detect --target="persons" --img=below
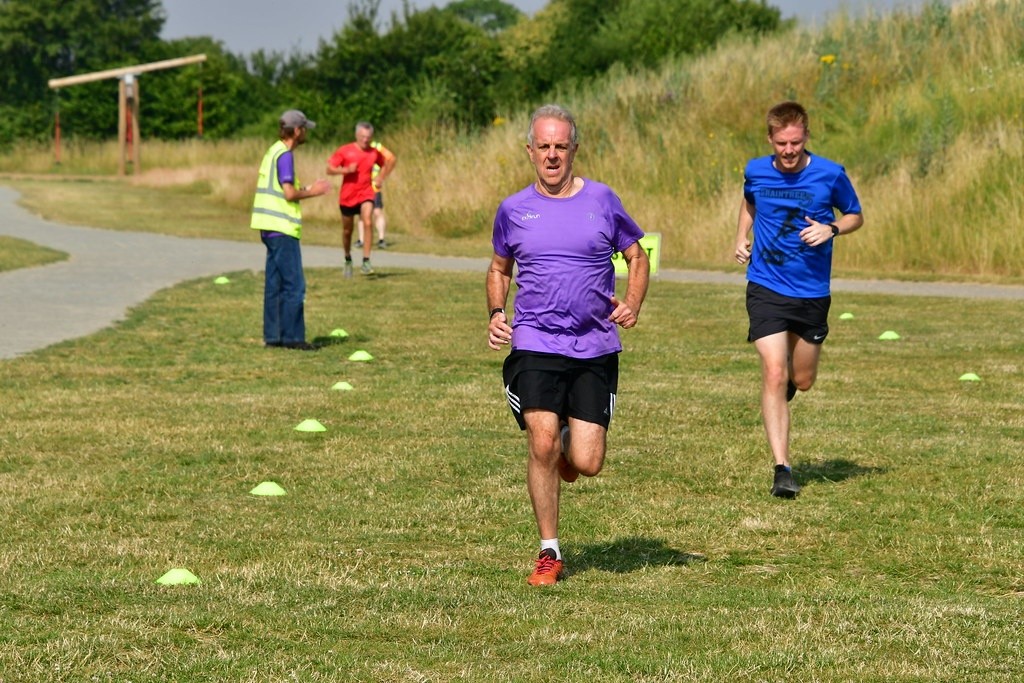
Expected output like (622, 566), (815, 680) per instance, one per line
(486, 104), (652, 587)
(734, 102), (865, 502)
(355, 141), (397, 248)
(326, 121), (386, 280)
(251, 110), (333, 352)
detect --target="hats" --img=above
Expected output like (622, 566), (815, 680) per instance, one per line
(280, 110), (315, 129)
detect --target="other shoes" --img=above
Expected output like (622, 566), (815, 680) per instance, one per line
(355, 240), (363, 247)
(360, 260), (374, 274)
(378, 239), (385, 249)
(282, 342), (321, 350)
(343, 261), (352, 279)
(266, 342), (282, 348)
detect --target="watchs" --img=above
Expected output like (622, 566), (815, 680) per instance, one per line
(827, 223), (839, 240)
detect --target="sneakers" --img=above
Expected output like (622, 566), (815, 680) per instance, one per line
(787, 378), (797, 401)
(528, 548), (563, 585)
(558, 419), (579, 482)
(770, 462), (799, 498)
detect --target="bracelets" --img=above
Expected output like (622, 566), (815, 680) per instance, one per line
(489, 307), (504, 320)
